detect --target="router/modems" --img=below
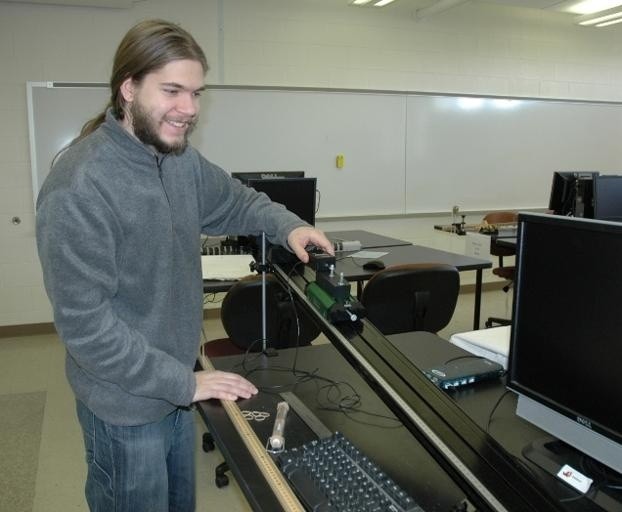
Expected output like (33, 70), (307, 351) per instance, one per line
(422, 358), (503, 391)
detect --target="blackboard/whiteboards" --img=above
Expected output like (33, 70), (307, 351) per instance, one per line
(24, 79), (622, 224)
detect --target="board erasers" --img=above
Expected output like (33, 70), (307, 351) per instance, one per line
(336, 153), (344, 170)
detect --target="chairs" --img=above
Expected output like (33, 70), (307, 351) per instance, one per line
(199, 275), (321, 361)
(362, 261), (460, 333)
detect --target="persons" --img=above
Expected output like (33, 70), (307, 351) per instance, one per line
(33, 17), (335, 511)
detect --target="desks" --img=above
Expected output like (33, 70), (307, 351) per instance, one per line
(307, 221), (514, 328)
(191, 328), (622, 512)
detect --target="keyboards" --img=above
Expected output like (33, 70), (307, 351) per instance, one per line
(273, 430), (425, 512)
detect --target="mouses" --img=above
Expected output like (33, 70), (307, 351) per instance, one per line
(363, 260), (386, 270)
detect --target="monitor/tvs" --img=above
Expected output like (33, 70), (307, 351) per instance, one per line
(504, 211), (622, 512)
(231, 172), (305, 189)
(592, 175), (622, 222)
(248, 176), (317, 229)
(548, 171), (600, 220)
